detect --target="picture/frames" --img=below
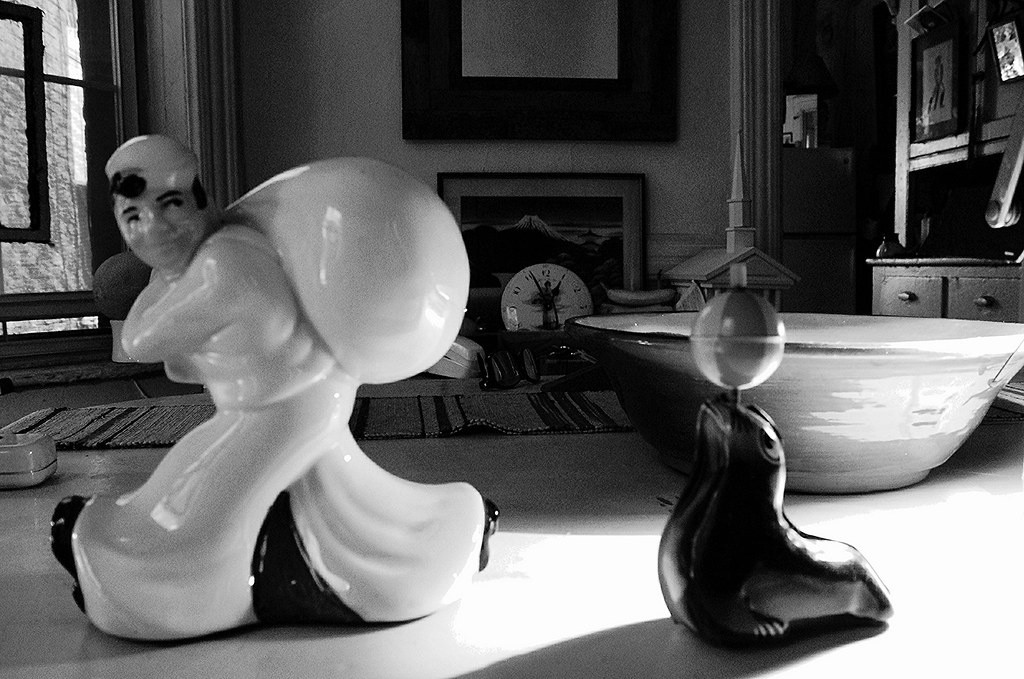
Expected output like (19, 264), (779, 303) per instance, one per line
(437, 170), (651, 338)
(908, 13), (973, 145)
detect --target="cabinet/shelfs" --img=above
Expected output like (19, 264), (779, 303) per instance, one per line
(866, 1), (1023, 384)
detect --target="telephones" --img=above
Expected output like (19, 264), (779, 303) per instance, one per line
(425, 335), (488, 379)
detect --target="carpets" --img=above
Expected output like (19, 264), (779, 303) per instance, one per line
(991, 380), (1023, 415)
(0, 390), (634, 453)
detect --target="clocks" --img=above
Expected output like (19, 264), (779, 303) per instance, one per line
(499, 262), (593, 331)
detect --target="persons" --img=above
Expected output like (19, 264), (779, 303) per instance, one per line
(48, 132), (503, 643)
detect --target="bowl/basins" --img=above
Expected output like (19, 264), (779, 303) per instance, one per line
(560, 309), (1024, 496)
